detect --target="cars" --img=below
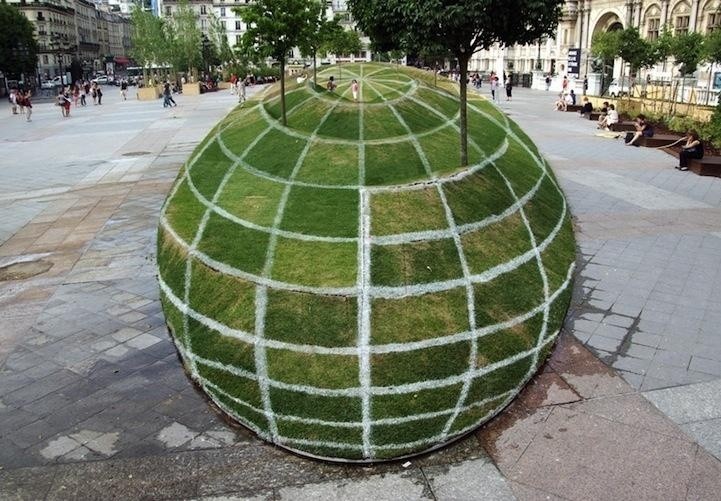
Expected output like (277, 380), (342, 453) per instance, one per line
(41, 73), (68, 88)
(90, 75), (107, 85)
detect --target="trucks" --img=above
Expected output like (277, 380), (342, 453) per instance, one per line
(608, 78), (633, 99)
(95, 70), (105, 78)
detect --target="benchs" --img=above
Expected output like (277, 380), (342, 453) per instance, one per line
(687, 154), (721, 175)
(638, 133), (686, 148)
(567, 105), (584, 112)
(590, 112), (630, 120)
(608, 122), (637, 131)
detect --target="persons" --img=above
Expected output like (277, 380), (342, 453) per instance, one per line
(2, 63), (48, 122)
(228, 72), (279, 102)
(583, 75), (589, 96)
(491, 77), (498, 99)
(504, 75), (513, 101)
(109, 73), (143, 100)
(580, 98), (593, 116)
(597, 102), (618, 131)
(55, 74), (102, 116)
(674, 128), (704, 171)
(326, 76), (359, 101)
(438, 64), (494, 88)
(626, 114), (654, 146)
(155, 72), (217, 108)
(554, 89), (576, 111)
(562, 76), (568, 90)
(546, 75), (549, 91)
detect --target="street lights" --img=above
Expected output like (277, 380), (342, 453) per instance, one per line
(48, 33), (71, 93)
(12, 40), (29, 83)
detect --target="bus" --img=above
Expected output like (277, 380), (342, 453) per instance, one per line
(127, 65), (174, 84)
